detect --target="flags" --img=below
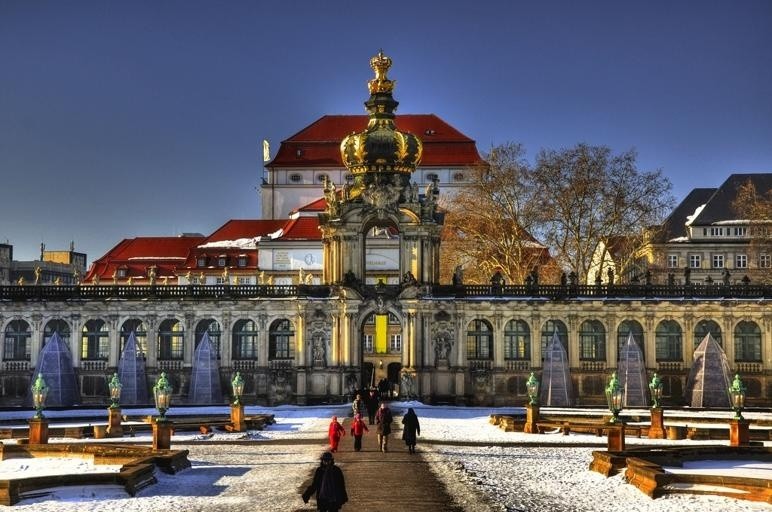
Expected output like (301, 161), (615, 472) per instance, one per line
(264, 139), (270, 161)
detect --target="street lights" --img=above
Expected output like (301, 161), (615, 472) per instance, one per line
(28, 372), (49, 443)
(648, 373), (666, 438)
(725, 374), (750, 446)
(523, 372), (543, 433)
(153, 372), (173, 449)
(106, 371), (126, 438)
(606, 372), (629, 454)
(229, 374), (247, 432)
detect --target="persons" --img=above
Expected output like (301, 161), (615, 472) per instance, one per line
(374, 400), (393, 453)
(350, 413), (369, 451)
(366, 390), (378, 425)
(451, 266), (750, 285)
(16, 266), (314, 287)
(352, 394), (365, 419)
(401, 407), (421, 453)
(302, 452), (348, 512)
(329, 415), (345, 451)
(324, 175), (435, 214)
